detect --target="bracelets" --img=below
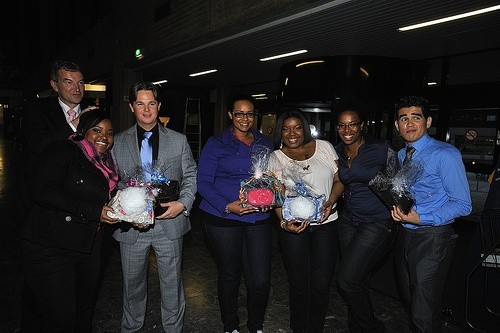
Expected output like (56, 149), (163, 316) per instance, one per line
(280, 220), (288, 229)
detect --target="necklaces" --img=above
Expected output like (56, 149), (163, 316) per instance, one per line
(287, 144), (309, 170)
(342, 134), (363, 160)
(233, 129), (249, 140)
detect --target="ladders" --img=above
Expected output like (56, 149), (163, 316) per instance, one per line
(184, 97), (202, 163)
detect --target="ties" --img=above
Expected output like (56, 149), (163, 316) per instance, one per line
(67, 108), (77, 122)
(139, 127), (153, 183)
(402, 146), (416, 167)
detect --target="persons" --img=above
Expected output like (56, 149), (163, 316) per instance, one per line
(106, 81), (197, 332)
(392, 95), (473, 332)
(19, 60), (103, 331)
(197, 93), (276, 333)
(265, 108), (346, 332)
(15, 108), (150, 332)
(333, 102), (402, 333)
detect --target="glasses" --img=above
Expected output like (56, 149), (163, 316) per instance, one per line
(337, 121), (361, 129)
(232, 112), (257, 118)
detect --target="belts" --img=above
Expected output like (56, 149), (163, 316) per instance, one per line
(401, 226), (453, 234)
